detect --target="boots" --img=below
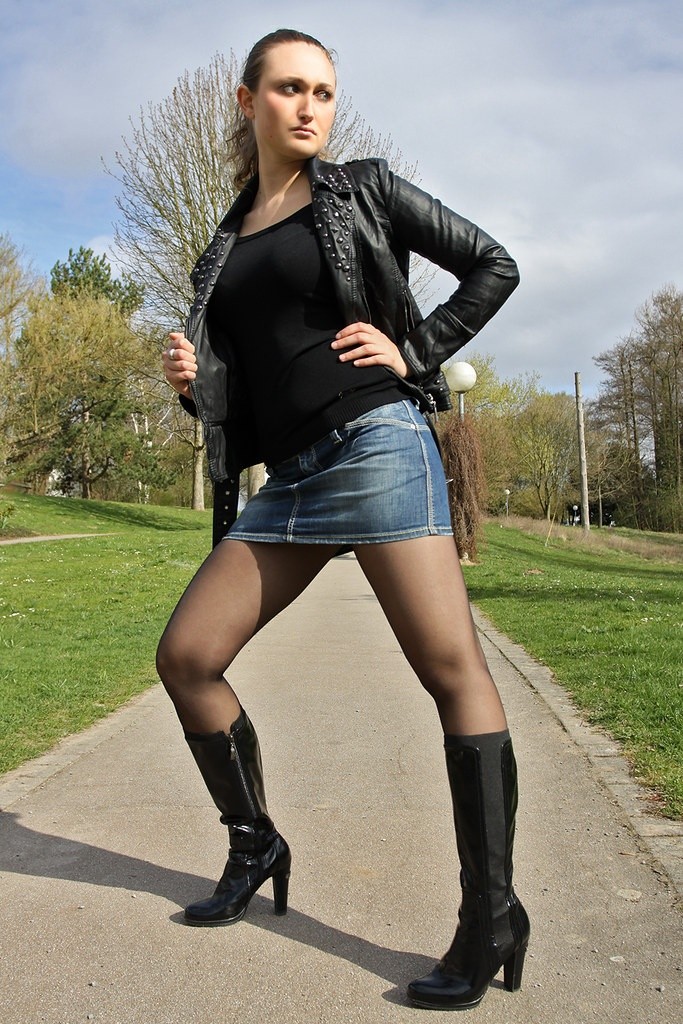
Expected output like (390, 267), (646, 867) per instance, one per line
(407, 729), (529, 1011)
(184, 705), (292, 926)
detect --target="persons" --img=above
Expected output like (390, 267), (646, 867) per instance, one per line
(155, 28), (530, 1011)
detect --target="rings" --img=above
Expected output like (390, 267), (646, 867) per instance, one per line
(168, 348), (175, 360)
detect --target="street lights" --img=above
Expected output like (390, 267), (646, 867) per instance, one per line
(445, 361), (478, 558)
(573, 505), (578, 524)
(505, 490), (510, 516)
